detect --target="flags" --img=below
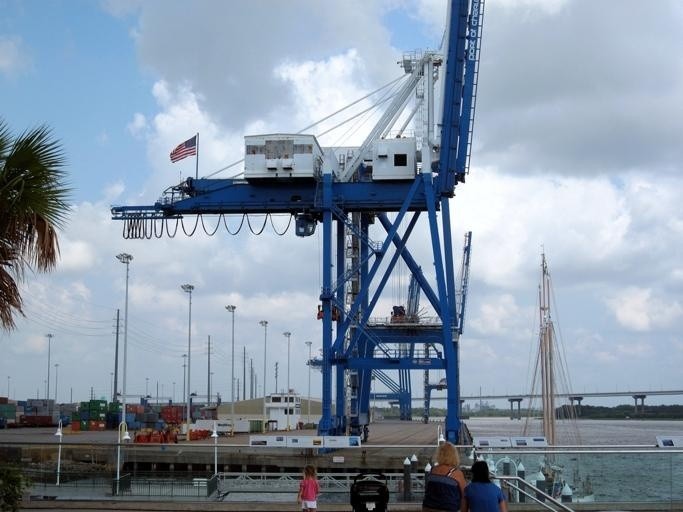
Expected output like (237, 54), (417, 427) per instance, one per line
(169, 135), (197, 164)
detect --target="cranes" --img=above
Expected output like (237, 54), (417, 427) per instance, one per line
(111, 0), (485, 454)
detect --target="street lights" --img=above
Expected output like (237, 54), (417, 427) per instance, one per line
(116, 421), (131, 496)
(284, 332), (291, 431)
(116, 254), (133, 445)
(305, 342), (312, 424)
(259, 320), (268, 433)
(7, 333), (214, 405)
(211, 421), (219, 474)
(182, 285), (194, 441)
(226, 305), (236, 413)
(438, 425), (446, 447)
(55, 419), (63, 486)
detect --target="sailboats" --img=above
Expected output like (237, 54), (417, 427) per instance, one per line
(522, 254), (594, 503)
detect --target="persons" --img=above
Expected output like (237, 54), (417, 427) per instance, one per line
(296, 466), (319, 511)
(420, 442), (470, 511)
(461, 461), (507, 511)
